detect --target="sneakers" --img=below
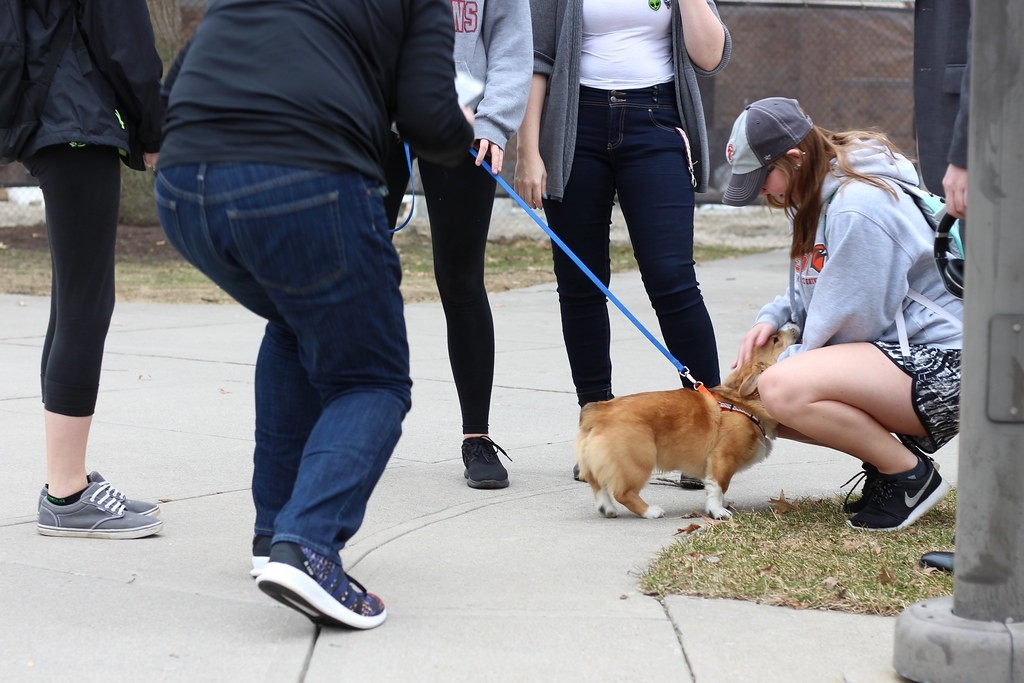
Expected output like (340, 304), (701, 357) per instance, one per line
(461, 435), (513, 489)
(250, 535), (385, 629)
(840, 442), (950, 532)
(36, 471), (163, 539)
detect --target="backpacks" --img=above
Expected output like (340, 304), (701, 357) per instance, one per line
(825, 174), (965, 259)
(0, 0), (76, 164)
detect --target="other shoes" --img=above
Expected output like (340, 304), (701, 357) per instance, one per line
(920, 551), (955, 576)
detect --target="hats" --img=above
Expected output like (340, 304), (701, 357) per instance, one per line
(722, 97), (813, 206)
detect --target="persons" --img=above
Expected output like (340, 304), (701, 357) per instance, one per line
(379, 0), (534, 489)
(905, 0), (975, 572)
(0, 0), (165, 545)
(513, 0), (733, 490)
(152, 1), (481, 631)
(722, 97), (963, 531)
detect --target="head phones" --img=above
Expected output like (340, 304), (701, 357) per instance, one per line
(934, 211), (966, 298)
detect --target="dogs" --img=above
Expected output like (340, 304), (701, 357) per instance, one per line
(574, 319), (802, 521)
